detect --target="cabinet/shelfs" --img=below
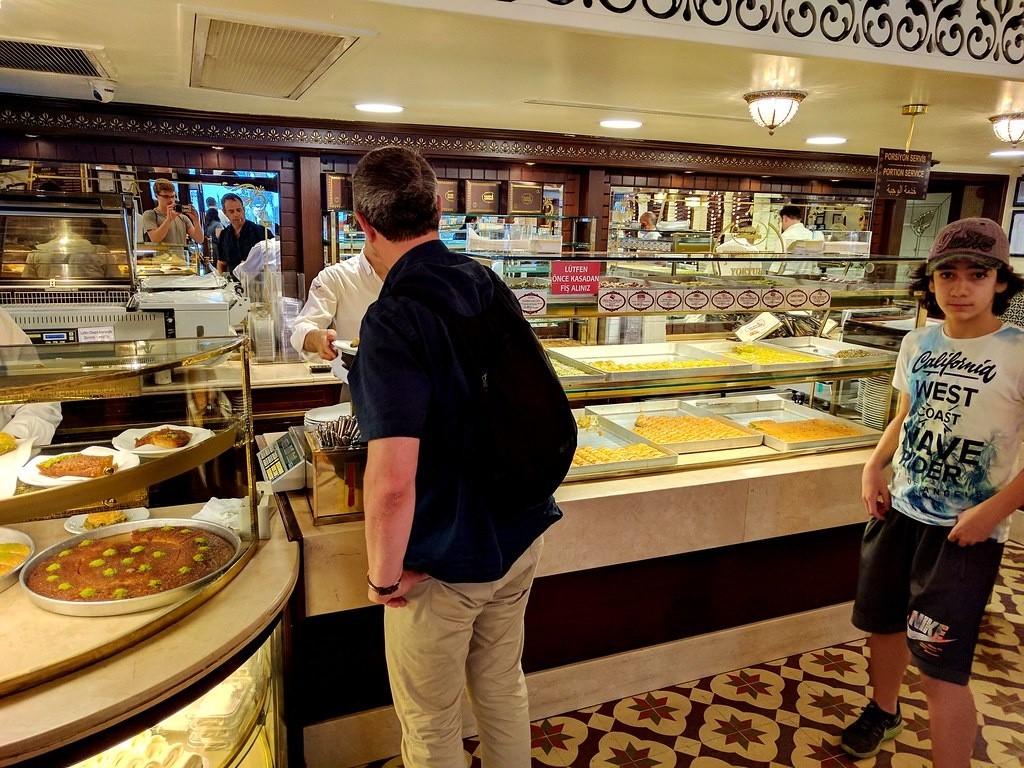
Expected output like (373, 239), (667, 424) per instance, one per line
(0, 335), (260, 700)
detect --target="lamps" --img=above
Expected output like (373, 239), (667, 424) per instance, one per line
(988, 112), (1024, 149)
(743, 89), (808, 137)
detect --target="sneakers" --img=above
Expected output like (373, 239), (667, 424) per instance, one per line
(840, 698), (905, 758)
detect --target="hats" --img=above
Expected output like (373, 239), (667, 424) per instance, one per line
(927, 216), (1009, 273)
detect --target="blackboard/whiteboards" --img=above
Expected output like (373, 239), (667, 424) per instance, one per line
(874, 148), (933, 201)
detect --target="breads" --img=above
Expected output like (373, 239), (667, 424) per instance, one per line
(27, 525), (235, 602)
(0, 543), (29, 576)
(134, 428), (190, 448)
(35, 453), (119, 478)
(566, 346), (870, 467)
(0, 431), (19, 456)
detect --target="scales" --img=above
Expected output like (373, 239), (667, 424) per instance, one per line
(256, 424), (328, 493)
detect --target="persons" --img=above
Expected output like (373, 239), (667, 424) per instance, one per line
(767, 205), (824, 276)
(0, 308), (63, 455)
(142, 178), (204, 266)
(21, 179), (122, 280)
(703, 215), (763, 276)
(850, 216), (871, 242)
(127, 207), (145, 244)
(635, 211), (671, 267)
(839, 216), (1024, 768)
(289, 234), (392, 361)
(348, 145), (581, 768)
(453, 214), (480, 240)
(489, 214), (513, 239)
(205, 193), (281, 287)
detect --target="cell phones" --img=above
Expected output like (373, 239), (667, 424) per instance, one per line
(175, 205), (191, 212)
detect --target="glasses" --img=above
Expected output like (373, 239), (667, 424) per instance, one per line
(158, 193), (175, 199)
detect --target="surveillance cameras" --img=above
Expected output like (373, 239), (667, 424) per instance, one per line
(88, 80), (114, 103)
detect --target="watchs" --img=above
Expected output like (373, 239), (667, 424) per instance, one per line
(366, 570), (404, 596)
